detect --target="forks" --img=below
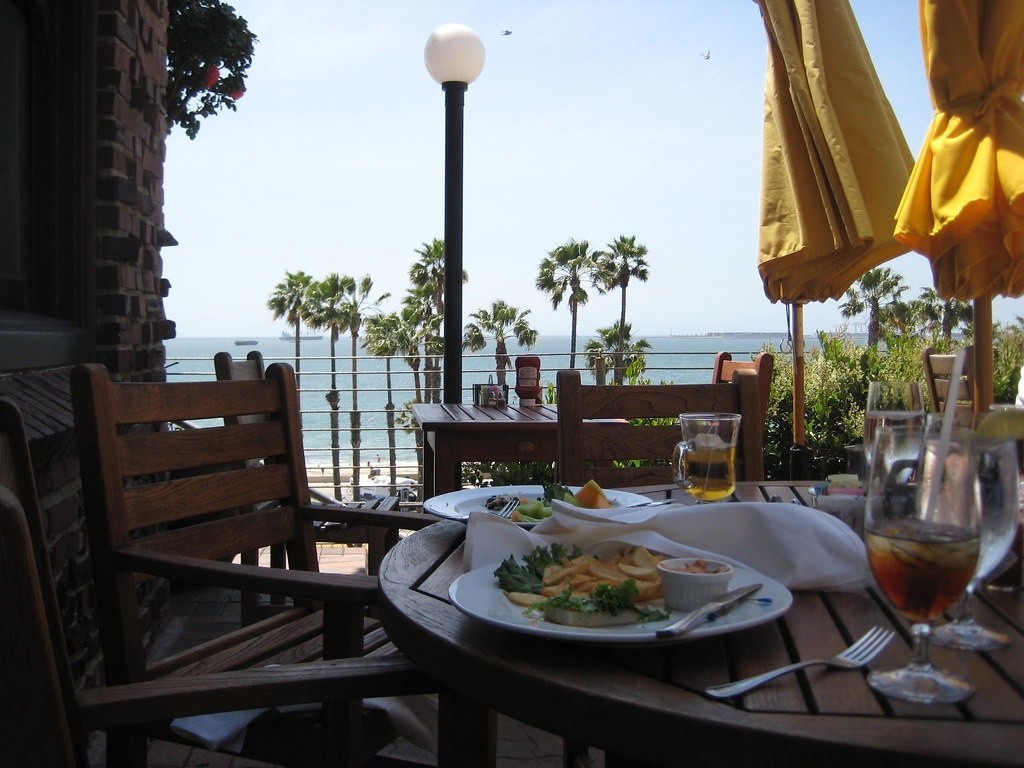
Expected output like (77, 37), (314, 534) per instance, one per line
(704, 625), (896, 698)
(466, 500), (520, 521)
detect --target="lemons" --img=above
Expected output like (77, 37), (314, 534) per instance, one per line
(976, 405), (1024, 443)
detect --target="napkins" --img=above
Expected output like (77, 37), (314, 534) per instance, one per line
(460, 498), (871, 601)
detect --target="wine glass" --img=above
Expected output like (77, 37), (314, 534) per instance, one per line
(862, 424), (981, 704)
(921, 440), (1018, 653)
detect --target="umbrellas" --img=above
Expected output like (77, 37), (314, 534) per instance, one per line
(893, 0), (1024, 412)
(760, 0), (916, 446)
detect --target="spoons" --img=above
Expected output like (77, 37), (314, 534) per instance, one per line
(769, 495), (783, 503)
(790, 499), (801, 505)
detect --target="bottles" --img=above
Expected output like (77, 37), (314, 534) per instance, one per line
(514, 357), (541, 406)
(480, 387), (505, 408)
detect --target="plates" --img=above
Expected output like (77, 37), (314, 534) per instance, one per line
(422, 485), (654, 527)
(449, 551), (794, 643)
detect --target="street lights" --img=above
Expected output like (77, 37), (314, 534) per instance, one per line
(422, 22), (486, 495)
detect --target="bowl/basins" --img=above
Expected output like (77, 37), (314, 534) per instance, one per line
(657, 558), (734, 612)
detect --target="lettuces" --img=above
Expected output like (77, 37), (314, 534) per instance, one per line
(495, 542), (584, 594)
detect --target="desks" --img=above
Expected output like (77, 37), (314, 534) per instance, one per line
(408, 404), (639, 498)
(378, 479), (1024, 768)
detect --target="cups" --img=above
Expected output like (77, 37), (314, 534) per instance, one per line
(864, 380), (926, 483)
(671, 411), (743, 505)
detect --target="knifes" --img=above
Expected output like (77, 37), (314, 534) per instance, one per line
(627, 498), (678, 507)
(656, 582), (763, 637)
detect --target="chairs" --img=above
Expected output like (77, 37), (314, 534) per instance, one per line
(0, 487), (497, 768)
(557, 351), (774, 484)
(73, 359), (437, 768)
(214, 351), (401, 623)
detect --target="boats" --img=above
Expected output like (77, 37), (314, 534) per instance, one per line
(280, 331), (324, 341)
